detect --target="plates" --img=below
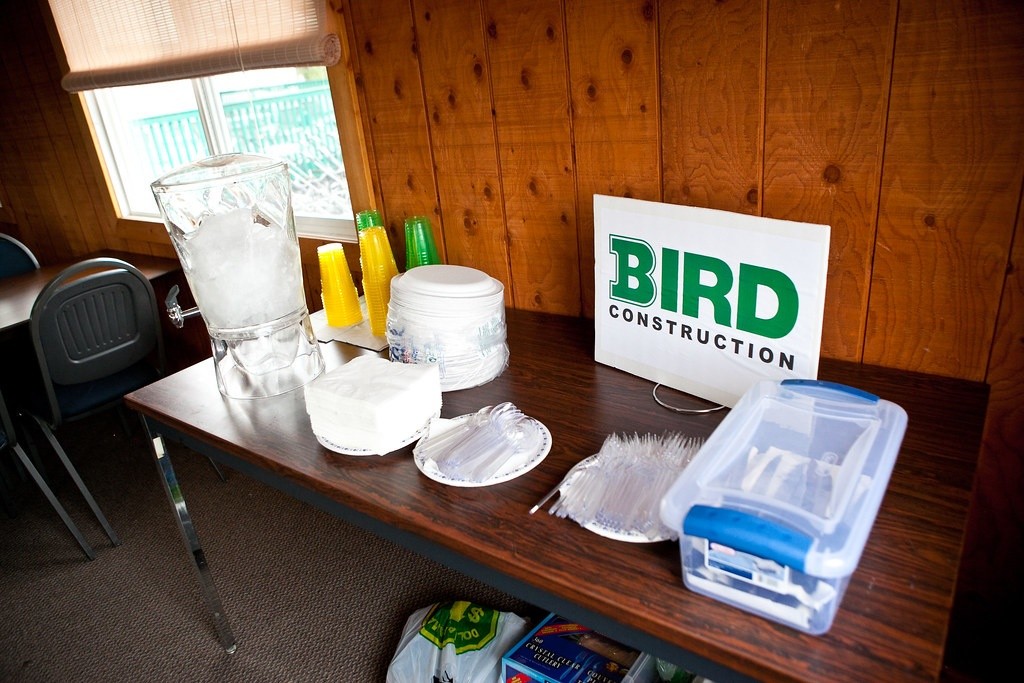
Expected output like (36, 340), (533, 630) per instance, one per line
(316, 411), (441, 456)
(415, 412), (552, 488)
(386, 265), (507, 393)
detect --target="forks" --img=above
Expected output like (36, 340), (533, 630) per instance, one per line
(530, 430), (704, 540)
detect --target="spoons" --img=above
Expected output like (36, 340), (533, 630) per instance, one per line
(412, 402), (538, 484)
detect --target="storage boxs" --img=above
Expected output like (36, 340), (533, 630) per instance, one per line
(659, 378), (909, 637)
(499, 611), (716, 681)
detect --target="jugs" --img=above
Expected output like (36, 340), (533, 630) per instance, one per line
(560, 454), (678, 542)
(150, 153), (325, 399)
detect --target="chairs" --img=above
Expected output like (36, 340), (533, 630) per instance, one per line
(10, 255), (226, 549)
(0, 388), (101, 562)
(0, 231), (41, 280)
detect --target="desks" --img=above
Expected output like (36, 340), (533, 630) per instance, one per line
(0, 247), (181, 343)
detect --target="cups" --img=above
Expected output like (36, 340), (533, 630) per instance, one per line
(317, 210), (442, 336)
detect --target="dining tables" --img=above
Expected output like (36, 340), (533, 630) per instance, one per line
(125, 293), (994, 683)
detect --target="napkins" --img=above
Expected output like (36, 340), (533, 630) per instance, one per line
(303, 353), (448, 456)
(424, 415), (537, 479)
